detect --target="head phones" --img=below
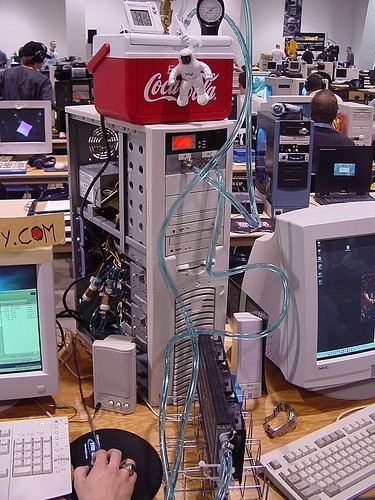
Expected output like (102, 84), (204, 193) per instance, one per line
(270, 103), (301, 116)
(19, 43), (46, 63)
(28, 155), (56, 169)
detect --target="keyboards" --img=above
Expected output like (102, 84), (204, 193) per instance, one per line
(255, 403), (375, 500)
(0, 415), (73, 500)
(0, 161), (27, 173)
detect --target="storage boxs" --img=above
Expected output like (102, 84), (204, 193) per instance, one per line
(86, 34), (233, 125)
(233, 148), (255, 163)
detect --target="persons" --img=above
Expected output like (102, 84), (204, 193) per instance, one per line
(0, 41), (55, 111)
(47, 41), (58, 66)
(316, 45), (341, 63)
(233, 56), (243, 72)
(314, 62), (332, 89)
(270, 45), (286, 72)
(236, 71), (266, 123)
(302, 45), (315, 76)
(345, 47), (354, 68)
(309, 89), (356, 192)
(285, 37), (298, 60)
(305, 75), (344, 104)
(74, 449), (137, 500)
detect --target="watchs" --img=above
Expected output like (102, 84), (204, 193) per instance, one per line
(263, 401), (297, 439)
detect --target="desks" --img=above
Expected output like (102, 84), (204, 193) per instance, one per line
(232, 164), (257, 192)
(0, 322), (375, 500)
(0, 155), (70, 200)
(52, 136), (67, 154)
(228, 192), (375, 246)
(0, 200), (74, 253)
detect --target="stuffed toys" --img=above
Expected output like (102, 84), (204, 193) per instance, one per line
(167, 49), (212, 106)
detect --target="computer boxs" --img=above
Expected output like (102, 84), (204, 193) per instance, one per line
(256, 111), (314, 208)
(65, 104), (234, 405)
(338, 101), (374, 147)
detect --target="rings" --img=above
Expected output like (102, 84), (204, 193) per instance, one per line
(122, 463), (136, 475)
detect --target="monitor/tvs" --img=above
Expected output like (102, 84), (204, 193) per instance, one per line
(0, 246), (60, 412)
(232, 57), (360, 101)
(240, 200), (375, 399)
(0, 100), (53, 162)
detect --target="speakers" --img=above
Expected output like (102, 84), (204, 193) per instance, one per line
(230, 311), (264, 399)
(92, 334), (138, 414)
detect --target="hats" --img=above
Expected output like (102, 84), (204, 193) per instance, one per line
(21, 41), (52, 59)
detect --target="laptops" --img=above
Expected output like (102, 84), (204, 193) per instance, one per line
(312, 146), (375, 205)
